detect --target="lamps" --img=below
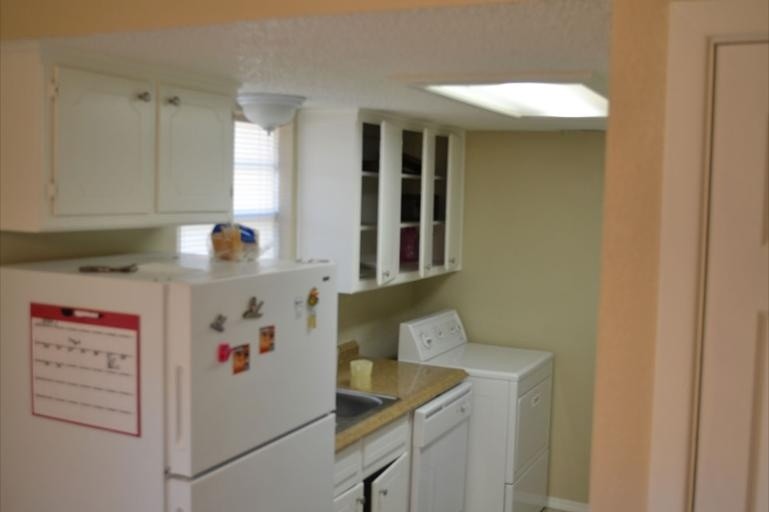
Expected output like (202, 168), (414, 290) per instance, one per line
(237, 93), (305, 132)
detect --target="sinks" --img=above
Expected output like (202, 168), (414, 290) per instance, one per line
(337, 387), (400, 434)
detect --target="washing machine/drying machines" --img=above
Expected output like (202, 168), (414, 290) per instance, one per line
(398, 312), (556, 510)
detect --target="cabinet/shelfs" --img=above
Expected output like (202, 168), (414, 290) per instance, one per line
(337, 414), (412, 512)
(412, 382), (470, 511)
(293, 111), (466, 291)
(0, 63), (237, 235)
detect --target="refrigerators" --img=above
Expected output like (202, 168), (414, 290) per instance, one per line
(0, 251), (340, 511)
